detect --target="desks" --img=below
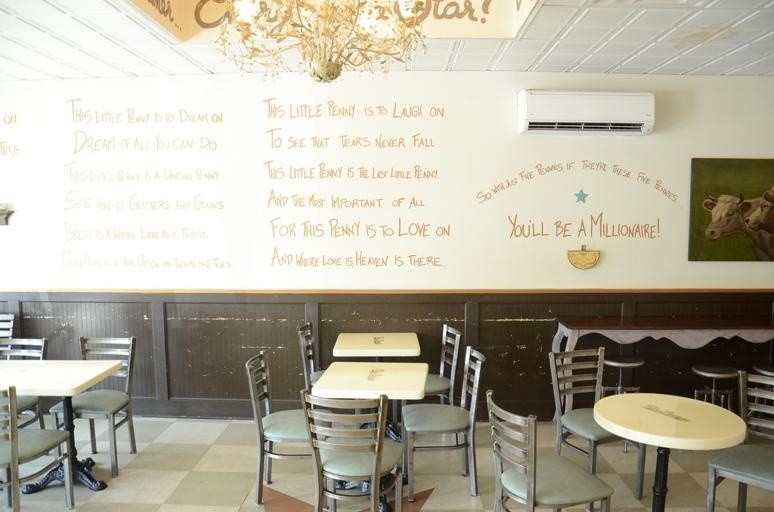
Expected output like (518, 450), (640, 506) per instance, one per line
(310, 361), (428, 446)
(592, 390), (747, 512)
(552, 316), (770, 427)
(0, 358), (122, 496)
(331, 331), (423, 359)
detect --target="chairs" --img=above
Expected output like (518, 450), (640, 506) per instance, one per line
(296, 322), (331, 404)
(422, 323), (464, 455)
(401, 345), (486, 503)
(244, 349), (332, 506)
(50, 336), (138, 480)
(1, 337), (47, 432)
(706, 370), (768, 509)
(300, 389), (405, 512)
(0, 313), (16, 360)
(0, 387), (74, 512)
(549, 347), (646, 500)
(487, 388), (614, 512)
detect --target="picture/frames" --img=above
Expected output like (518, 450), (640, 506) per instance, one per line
(689, 157), (774, 264)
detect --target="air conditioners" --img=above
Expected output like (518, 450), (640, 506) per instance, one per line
(518, 86), (657, 136)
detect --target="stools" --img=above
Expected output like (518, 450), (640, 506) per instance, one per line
(689, 361), (742, 418)
(603, 352), (648, 453)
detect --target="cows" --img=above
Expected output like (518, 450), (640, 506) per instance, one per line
(745, 186), (774, 248)
(702, 193), (774, 262)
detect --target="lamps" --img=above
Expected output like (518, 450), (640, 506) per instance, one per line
(214, 0), (431, 86)
